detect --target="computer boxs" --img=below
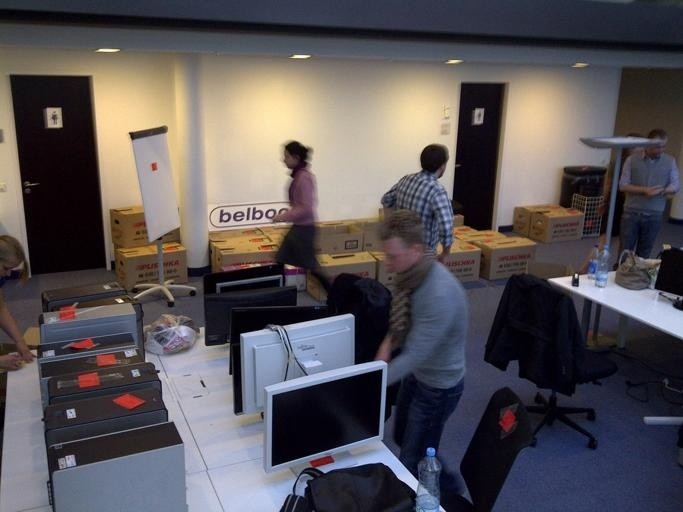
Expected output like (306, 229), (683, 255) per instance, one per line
(41, 281), (144, 359)
(36, 331), (186, 512)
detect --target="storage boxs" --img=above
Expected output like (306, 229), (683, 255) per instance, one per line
(531, 210), (586, 245)
(284, 263), (308, 292)
(216, 242), (282, 266)
(115, 241), (189, 295)
(379, 207), (387, 221)
(314, 251), (376, 292)
(266, 231), (290, 250)
(455, 230), (509, 244)
(514, 203), (562, 238)
(259, 225), (294, 236)
(208, 228), (265, 273)
(438, 243), (482, 285)
(220, 263), (279, 272)
(312, 223), (364, 251)
(473, 237), (537, 282)
(112, 206), (182, 247)
(369, 247), (406, 289)
(453, 213), (466, 226)
(453, 225), (473, 231)
(356, 216), (387, 252)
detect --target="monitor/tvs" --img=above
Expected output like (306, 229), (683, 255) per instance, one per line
(264, 359), (389, 482)
(203, 263), (298, 348)
(654, 247), (683, 298)
(228, 305), (358, 417)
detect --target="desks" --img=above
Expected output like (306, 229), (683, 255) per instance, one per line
(551, 266), (683, 427)
(6, 327), (443, 507)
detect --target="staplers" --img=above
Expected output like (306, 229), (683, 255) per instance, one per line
(572, 272), (579, 286)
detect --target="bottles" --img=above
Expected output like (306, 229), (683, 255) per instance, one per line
(413, 446), (443, 512)
(589, 244), (599, 280)
(595, 244), (610, 288)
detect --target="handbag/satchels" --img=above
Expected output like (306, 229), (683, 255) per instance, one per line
(280, 461), (417, 511)
(614, 249), (656, 290)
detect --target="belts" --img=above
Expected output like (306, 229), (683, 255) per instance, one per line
(623, 207), (663, 218)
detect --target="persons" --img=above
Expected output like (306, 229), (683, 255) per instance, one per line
(0, 236), (37, 370)
(373, 209), (468, 481)
(273, 141), (333, 293)
(381, 143), (454, 264)
(613, 128), (680, 272)
(577, 134), (644, 276)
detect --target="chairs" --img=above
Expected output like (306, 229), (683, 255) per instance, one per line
(411, 389), (534, 507)
(507, 274), (618, 450)
